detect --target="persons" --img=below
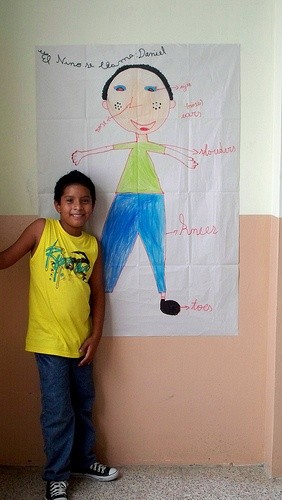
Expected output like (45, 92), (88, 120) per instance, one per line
(0, 170), (120, 500)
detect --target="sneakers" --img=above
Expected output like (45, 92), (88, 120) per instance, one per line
(70, 461), (119, 482)
(43, 480), (69, 500)
